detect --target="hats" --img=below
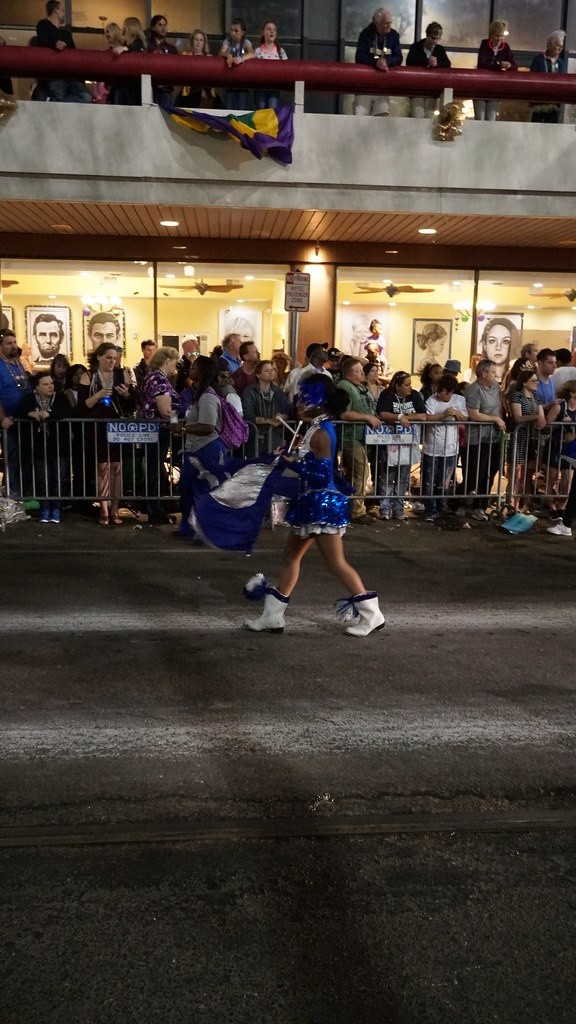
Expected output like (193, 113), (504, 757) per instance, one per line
(328, 348), (340, 359)
(306, 342), (329, 358)
(444, 359), (461, 373)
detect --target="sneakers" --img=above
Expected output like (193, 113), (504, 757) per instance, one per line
(546, 521), (572, 536)
(49, 509), (61, 523)
(40, 510), (50, 522)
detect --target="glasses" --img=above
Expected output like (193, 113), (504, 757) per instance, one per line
(527, 380), (540, 385)
(315, 348), (326, 351)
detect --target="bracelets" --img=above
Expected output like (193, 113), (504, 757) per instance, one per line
(398, 413), (404, 420)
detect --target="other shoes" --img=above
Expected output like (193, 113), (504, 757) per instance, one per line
(550, 512), (563, 521)
(148, 514), (176, 524)
(353, 514), (377, 523)
(380, 514), (390, 519)
(394, 515), (409, 519)
(472, 508), (489, 521)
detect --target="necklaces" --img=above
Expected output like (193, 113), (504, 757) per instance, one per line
(88, 371), (113, 403)
(489, 38), (505, 52)
(373, 32), (387, 59)
(311, 413), (332, 425)
(0, 354), (28, 390)
(396, 394), (406, 414)
(34, 392), (50, 411)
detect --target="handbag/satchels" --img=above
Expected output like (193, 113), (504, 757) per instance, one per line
(388, 425), (421, 466)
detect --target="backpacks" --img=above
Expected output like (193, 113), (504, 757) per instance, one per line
(195, 389), (249, 448)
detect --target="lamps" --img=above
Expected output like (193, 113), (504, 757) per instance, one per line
(353, 283), (435, 298)
(158, 279), (243, 295)
(452, 297), (496, 317)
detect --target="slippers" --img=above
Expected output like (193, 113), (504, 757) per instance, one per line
(99, 515), (110, 526)
(112, 515), (124, 525)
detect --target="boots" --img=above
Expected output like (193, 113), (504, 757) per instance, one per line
(243, 573), (291, 635)
(334, 591), (385, 637)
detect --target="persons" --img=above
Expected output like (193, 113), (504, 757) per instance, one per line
(143, 15), (179, 104)
(254, 21), (288, 109)
(103, 16), (148, 106)
(353, 7), (403, 117)
(226, 316), (255, 342)
(218, 18), (254, 110)
(33, 313), (65, 363)
(95, 23), (121, 104)
(528, 30), (566, 123)
(458, 343), (576, 536)
(419, 359), (470, 521)
(472, 19), (518, 121)
(406, 21), (451, 118)
(244, 373), (386, 637)
(0, 0), (94, 103)
(88, 313), (121, 353)
(349, 313), (371, 359)
(284, 341), (427, 519)
(416, 322), (448, 373)
(0, 328), (301, 526)
(175, 29), (224, 109)
(478, 318), (520, 385)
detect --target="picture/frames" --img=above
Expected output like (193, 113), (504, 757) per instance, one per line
(1, 305), (15, 332)
(411, 318), (453, 376)
(82, 307), (127, 359)
(475, 312), (523, 385)
(24, 305), (73, 372)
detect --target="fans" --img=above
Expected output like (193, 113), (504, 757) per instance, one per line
(527, 287), (576, 303)
